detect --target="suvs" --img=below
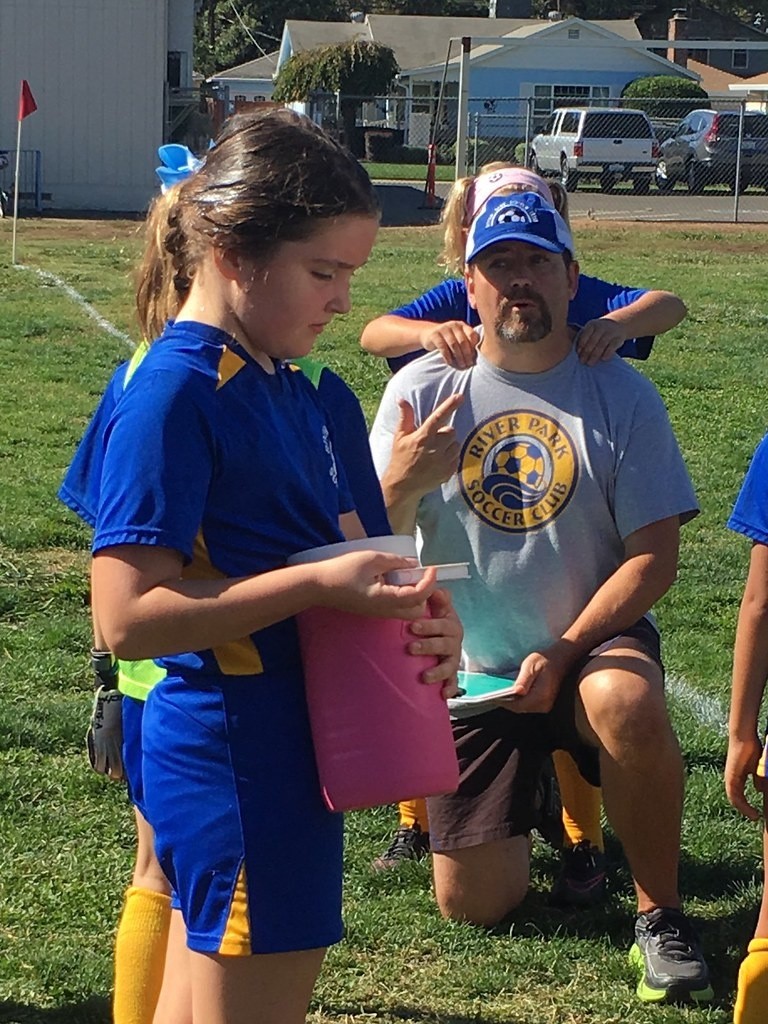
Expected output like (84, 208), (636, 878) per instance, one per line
(655, 108), (768, 193)
(529, 105), (660, 195)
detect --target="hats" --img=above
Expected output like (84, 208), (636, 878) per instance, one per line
(465, 191), (574, 265)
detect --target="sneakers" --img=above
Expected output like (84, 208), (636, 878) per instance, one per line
(375, 827), (431, 873)
(549, 836), (614, 903)
(629, 906), (720, 1005)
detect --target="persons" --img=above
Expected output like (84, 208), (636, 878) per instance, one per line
(53, 185), (398, 1024)
(725, 432), (768, 1023)
(93, 104), (465, 1024)
(366, 194), (714, 996)
(364, 161), (690, 903)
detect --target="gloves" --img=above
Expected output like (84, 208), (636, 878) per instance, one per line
(84, 645), (127, 782)
